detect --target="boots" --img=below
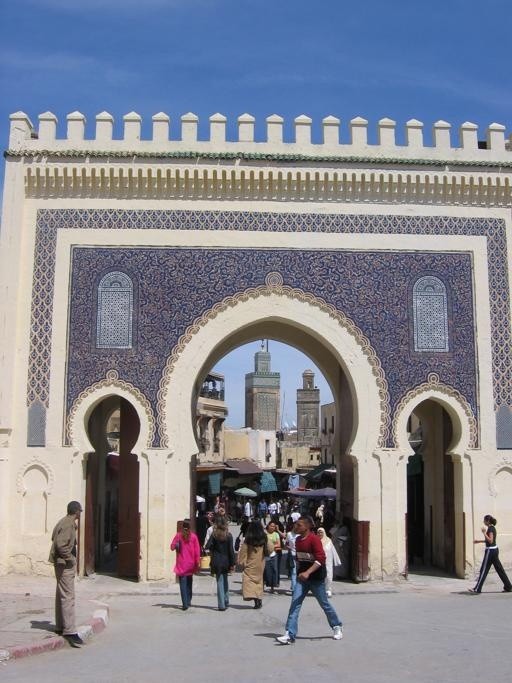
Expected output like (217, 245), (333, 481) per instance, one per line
(254, 598), (262, 609)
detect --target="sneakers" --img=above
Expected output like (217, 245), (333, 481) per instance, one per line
(63, 634), (83, 644)
(276, 631), (291, 645)
(332, 626), (342, 640)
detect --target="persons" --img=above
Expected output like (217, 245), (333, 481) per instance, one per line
(170, 519), (200, 611)
(275, 516), (343, 645)
(197, 498), (342, 610)
(466, 515), (512, 595)
(48, 501), (84, 645)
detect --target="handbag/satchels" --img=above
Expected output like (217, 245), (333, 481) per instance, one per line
(199, 556), (211, 569)
(286, 549), (295, 569)
(176, 543), (179, 552)
(235, 537), (240, 551)
(263, 536), (269, 557)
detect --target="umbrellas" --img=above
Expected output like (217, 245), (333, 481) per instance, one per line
(282, 486), (336, 501)
(235, 486), (258, 505)
(197, 493), (206, 502)
